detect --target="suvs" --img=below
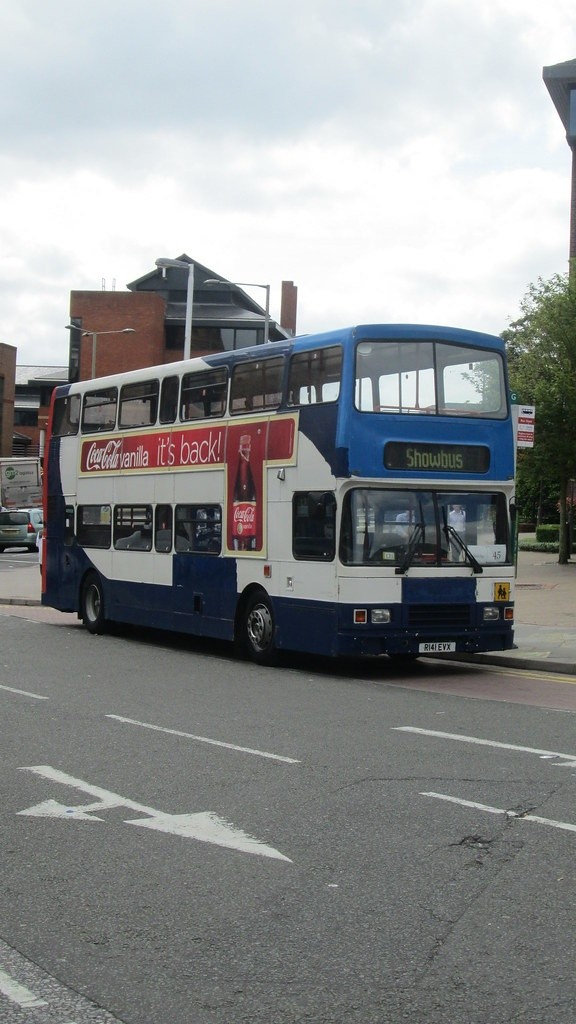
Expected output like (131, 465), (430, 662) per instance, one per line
(0, 507), (43, 553)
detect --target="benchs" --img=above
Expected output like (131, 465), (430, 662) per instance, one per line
(126, 544), (212, 552)
(67, 426), (111, 434)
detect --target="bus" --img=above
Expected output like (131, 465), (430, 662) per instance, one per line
(41, 322), (524, 670)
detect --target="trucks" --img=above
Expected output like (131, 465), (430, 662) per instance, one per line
(0, 456), (41, 511)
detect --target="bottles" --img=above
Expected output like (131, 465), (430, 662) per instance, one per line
(232, 436), (258, 550)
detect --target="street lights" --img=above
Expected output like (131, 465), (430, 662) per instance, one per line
(203, 278), (271, 344)
(65, 324), (137, 380)
(155, 257), (195, 360)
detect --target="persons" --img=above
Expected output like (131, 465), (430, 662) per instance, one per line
(396, 509), (415, 542)
(448, 504), (466, 562)
